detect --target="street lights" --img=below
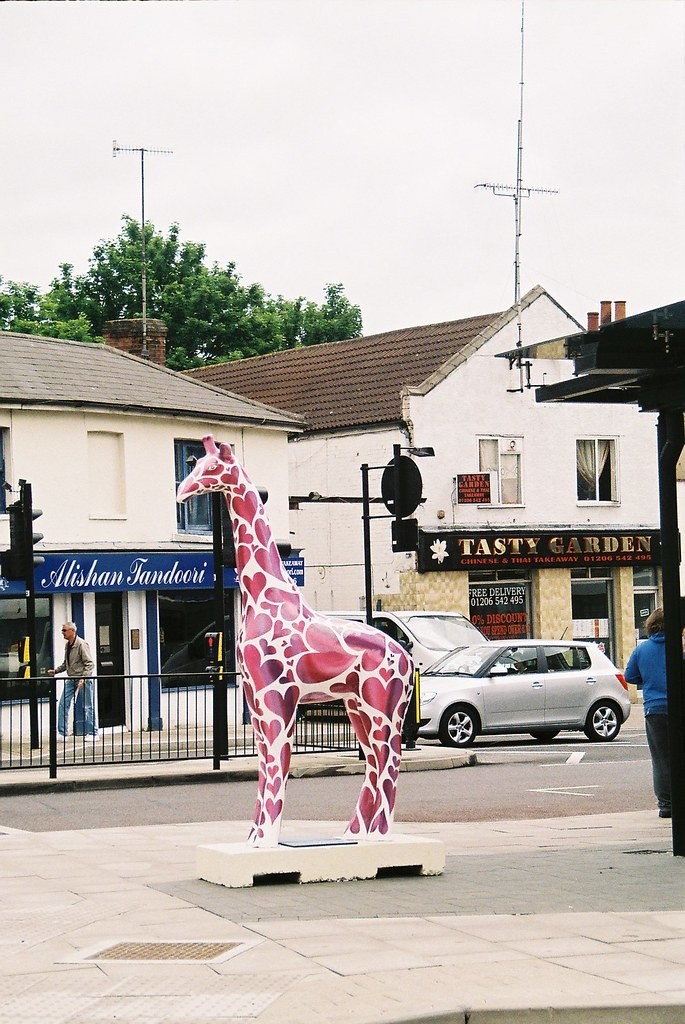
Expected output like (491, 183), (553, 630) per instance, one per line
(360, 443), (436, 628)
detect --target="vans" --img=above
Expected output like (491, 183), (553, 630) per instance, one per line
(162, 615), (233, 688)
(313, 608), (508, 677)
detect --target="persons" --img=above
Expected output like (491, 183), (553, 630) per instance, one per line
(48, 622), (100, 742)
(624, 607), (671, 817)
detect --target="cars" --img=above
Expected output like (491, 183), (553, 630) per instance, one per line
(401, 638), (632, 748)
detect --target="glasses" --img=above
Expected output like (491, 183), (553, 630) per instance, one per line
(61, 629), (69, 632)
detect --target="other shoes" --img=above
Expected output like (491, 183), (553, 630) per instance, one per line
(56, 732), (69, 743)
(83, 733), (100, 742)
(658, 809), (672, 819)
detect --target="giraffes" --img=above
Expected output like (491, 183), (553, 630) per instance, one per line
(176, 434), (416, 848)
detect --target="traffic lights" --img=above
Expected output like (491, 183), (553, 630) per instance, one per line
(222, 486), (269, 569)
(31, 508), (45, 569)
